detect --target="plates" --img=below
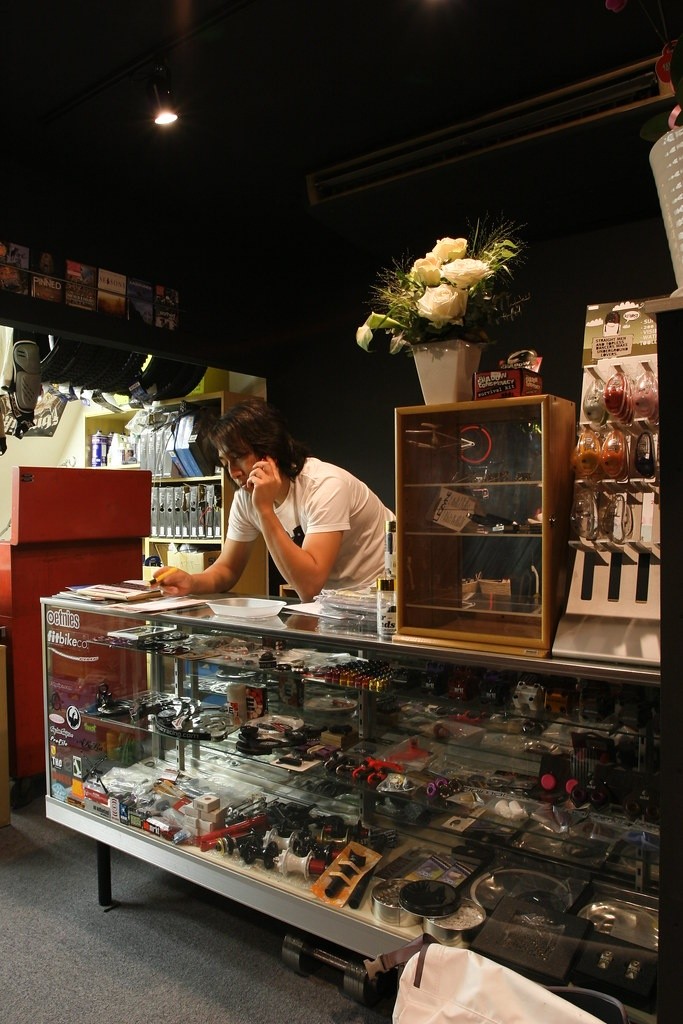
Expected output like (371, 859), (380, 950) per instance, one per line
(205, 596), (287, 619)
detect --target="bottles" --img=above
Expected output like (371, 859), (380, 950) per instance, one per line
(377, 521), (398, 637)
(90, 430), (131, 467)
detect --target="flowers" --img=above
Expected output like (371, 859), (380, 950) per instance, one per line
(356, 215), (529, 364)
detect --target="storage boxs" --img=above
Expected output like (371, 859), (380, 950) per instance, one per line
(143, 543), (177, 581)
(167, 550), (221, 575)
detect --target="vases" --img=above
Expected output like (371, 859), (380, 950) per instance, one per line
(411, 340), (480, 406)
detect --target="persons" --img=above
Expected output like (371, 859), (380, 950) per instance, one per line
(277, 614), (368, 666)
(148, 398), (395, 602)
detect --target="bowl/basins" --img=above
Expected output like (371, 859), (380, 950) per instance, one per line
(422, 898), (486, 950)
(370, 878), (423, 927)
(577, 901), (658, 953)
(469, 869), (572, 913)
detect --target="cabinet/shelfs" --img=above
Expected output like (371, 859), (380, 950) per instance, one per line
(41, 586), (661, 1024)
(392, 394), (577, 660)
(83, 389), (267, 597)
